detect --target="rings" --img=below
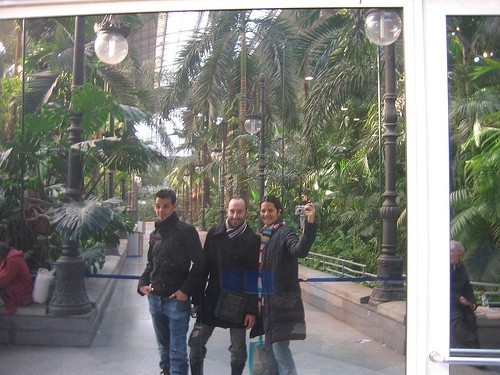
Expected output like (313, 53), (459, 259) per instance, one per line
(172, 294), (175, 296)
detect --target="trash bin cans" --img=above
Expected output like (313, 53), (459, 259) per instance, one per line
(137, 221), (145, 234)
(127, 232), (142, 257)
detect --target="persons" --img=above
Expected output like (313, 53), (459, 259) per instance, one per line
(188, 195), (258, 375)
(0, 242), (32, 312)
(450, 240), (485, 369)
(137, 189), (203, 375)
(259, 196), (317, 375)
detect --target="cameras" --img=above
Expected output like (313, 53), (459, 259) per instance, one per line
(295, 205), (306, 216)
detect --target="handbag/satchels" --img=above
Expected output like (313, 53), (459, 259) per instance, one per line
(249, 335), (278, 375)
(33, 268), (55, 303)
(214, 289), (247, 323)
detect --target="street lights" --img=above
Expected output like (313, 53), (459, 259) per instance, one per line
(132, 169), (141, 212)
(358, 9), (408, 303)
(212, 126), (226, 222)
(244, 73), (266, 231)
(44, 12), (131, 314)
(183, 168), (194, 226)
(195, 147), (206, 230)
